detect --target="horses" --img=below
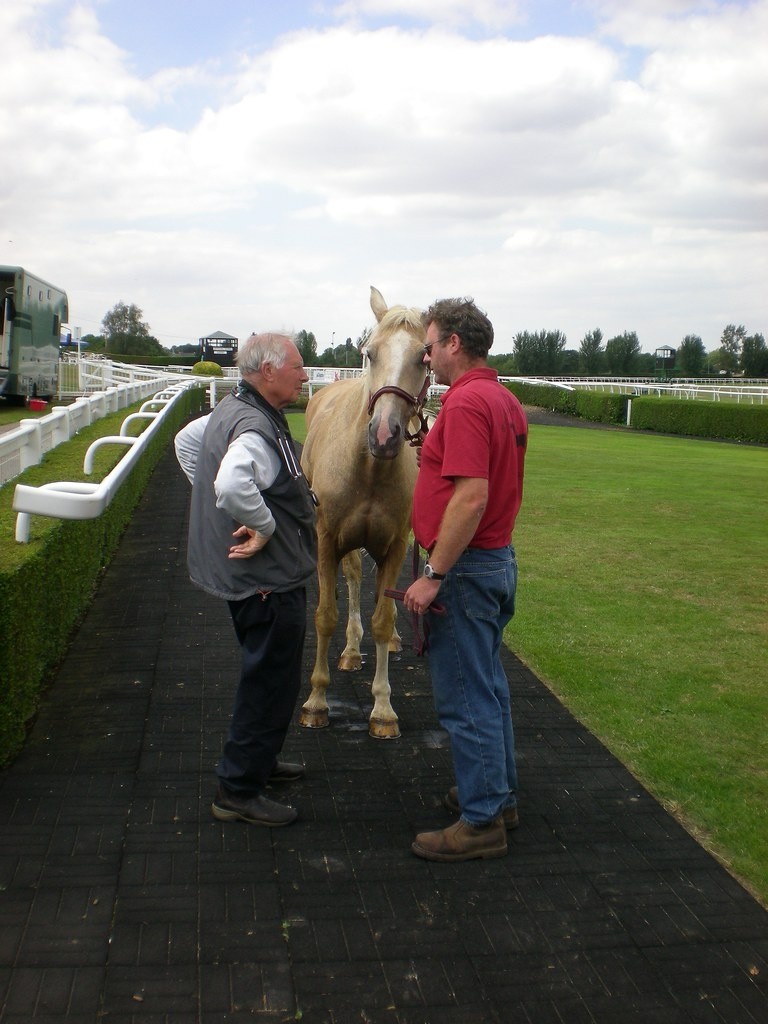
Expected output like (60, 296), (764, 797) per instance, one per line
(299, 285), (432, 740)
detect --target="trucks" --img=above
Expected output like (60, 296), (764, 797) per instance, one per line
(0, 264), (71, 408)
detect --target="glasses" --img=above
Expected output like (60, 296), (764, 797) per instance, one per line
(423, 332), (464, 357)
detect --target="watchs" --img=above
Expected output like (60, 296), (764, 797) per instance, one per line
(423, 560), (446, 580)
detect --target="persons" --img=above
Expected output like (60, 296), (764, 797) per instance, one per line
(404, 297), (528, 862)
(174, 333), (321, 831)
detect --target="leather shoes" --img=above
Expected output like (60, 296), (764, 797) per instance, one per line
(212, 782), (297, 826)
(445, 786), (519, 829)
(411, 816), (507, 862)
(267, 761), (305, 781)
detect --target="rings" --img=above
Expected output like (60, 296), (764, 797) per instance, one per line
(414, 608), (418, 612)
